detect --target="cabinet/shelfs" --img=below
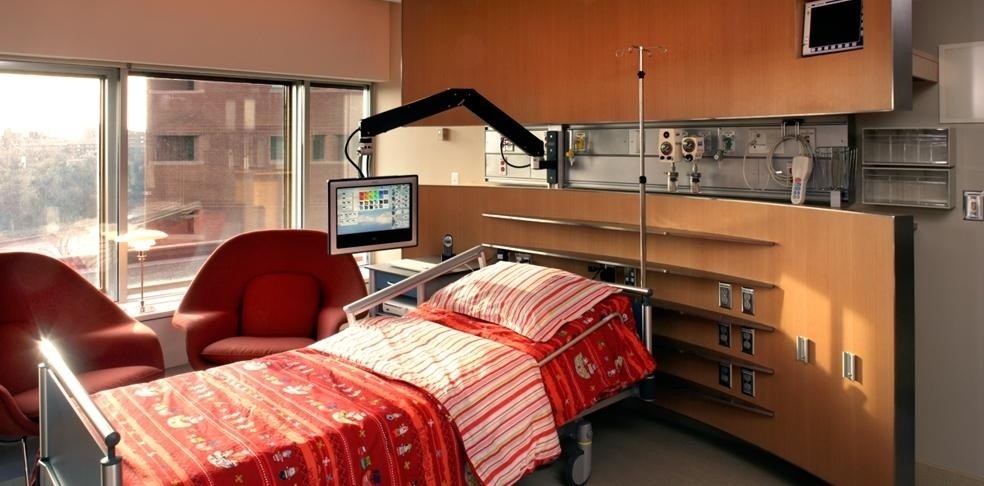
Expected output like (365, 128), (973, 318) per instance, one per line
(362, 257), (479, 322)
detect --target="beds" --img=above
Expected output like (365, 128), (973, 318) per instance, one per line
(30, 245), (652, 486)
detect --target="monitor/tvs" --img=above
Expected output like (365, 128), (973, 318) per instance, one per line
(802, 0), (864, 56)
(328, 175), (418, 256)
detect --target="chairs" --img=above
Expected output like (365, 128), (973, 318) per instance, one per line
(0, 252), (166, 485)
(168, 229), (369, 371)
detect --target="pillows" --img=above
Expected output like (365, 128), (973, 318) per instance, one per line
(238, 272), (322, 340)
(422, 257), (624, 344)
(0, 322), (50, 396)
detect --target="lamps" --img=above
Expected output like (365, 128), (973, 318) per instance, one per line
(108, 228), (168, 313)
